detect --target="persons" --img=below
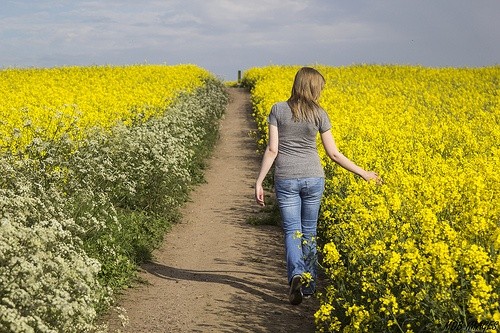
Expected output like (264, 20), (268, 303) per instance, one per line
(255, 67), (380, 306)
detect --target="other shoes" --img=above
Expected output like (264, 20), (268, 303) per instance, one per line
(304, 288), (317, 296)
(289, 274), (303, 306)
